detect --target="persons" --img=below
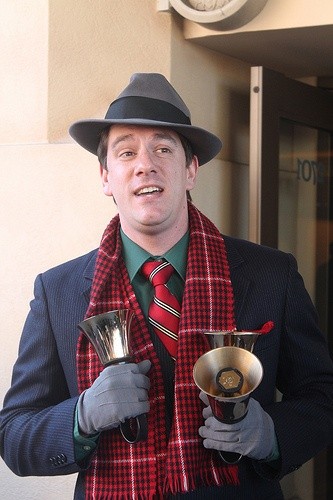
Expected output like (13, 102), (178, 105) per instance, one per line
(1, 74), (333, 500)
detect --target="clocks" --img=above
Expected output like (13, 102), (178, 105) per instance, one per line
(169, 0), (248, 24)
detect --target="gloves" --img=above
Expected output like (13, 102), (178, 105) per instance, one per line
(198, 394), (278, 460)
(78, 362), (159, 434)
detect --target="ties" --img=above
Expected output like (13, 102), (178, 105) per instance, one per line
(143, 259), (180, 362)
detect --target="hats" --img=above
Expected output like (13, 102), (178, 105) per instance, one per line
(69, 70), (222, 166)
